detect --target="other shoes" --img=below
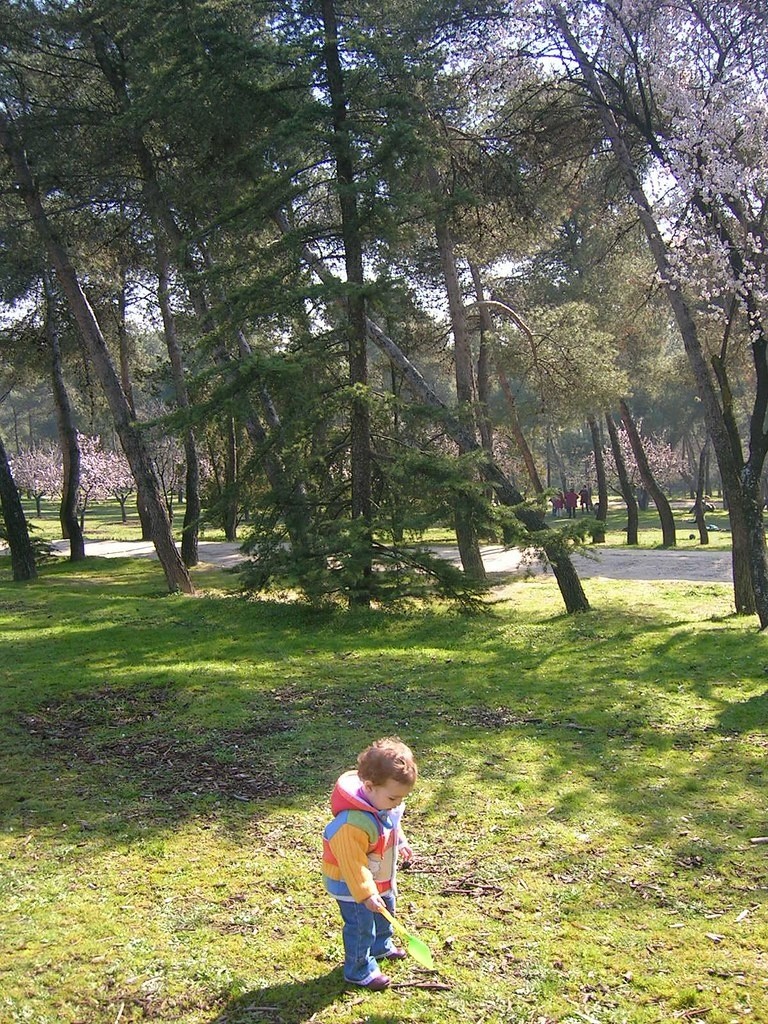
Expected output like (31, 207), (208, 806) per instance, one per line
(377, 948), (407, 960)
(366, 974), (391, 990)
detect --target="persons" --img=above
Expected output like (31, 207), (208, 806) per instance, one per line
(578, 485), (591, 515)
(322, 739), (417, 989)
(549, 488), (579, 518)
(687, 495), (716, 523)
